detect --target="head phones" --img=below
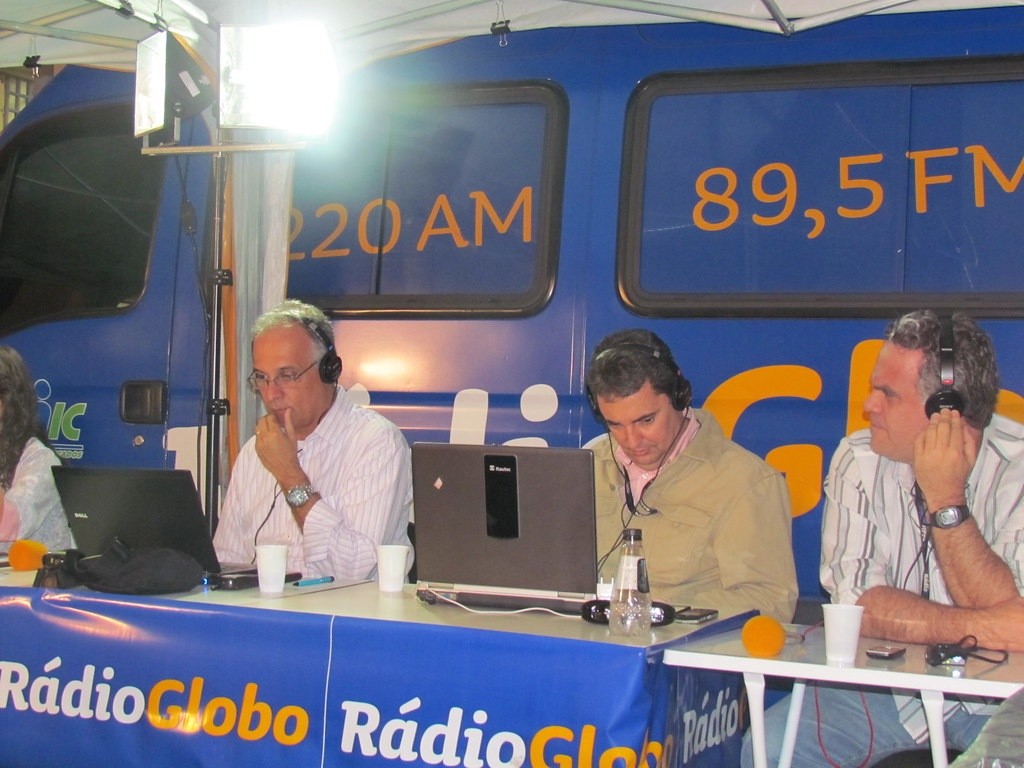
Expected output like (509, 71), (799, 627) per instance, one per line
(923, 322), (966, 422)
(586, 345), (691, 423)
(297, 316), (343, 384)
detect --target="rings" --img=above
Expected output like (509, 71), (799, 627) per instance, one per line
(255, 431), (260, 435)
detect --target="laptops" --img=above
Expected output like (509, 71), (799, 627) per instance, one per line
(50, 465), (260, 584)
(411, 441), (611, 616)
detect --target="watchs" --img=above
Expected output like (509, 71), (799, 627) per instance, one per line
(929, 504), (969, 529)
(285, 484), (317, 507)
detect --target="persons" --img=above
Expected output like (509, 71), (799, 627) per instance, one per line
(212, 299), (415, 583)
(741, 310), (1024, 768)
(586, 329), (799, 623)
(0, 346), (77, 549)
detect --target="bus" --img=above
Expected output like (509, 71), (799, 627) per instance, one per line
(0, 7), (1022, 596)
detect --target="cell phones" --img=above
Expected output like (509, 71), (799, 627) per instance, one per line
(674, 607), (719, 624)
(867, 646), (906, 659)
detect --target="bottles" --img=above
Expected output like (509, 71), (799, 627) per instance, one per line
(609, 527), (654, 639)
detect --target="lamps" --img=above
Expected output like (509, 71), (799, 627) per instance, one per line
(133, 23), (217, 148)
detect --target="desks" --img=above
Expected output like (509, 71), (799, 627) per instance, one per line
(0, 577), (1024, 768)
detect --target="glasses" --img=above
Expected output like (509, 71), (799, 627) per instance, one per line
(247, 361), (318, 390)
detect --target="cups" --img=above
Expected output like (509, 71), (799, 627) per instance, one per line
(255, 545), (287, 594)
(376, 545), (411, 592)
(821, 603), (865, 663)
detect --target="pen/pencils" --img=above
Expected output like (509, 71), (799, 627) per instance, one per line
(293, 577), (334, 586)
